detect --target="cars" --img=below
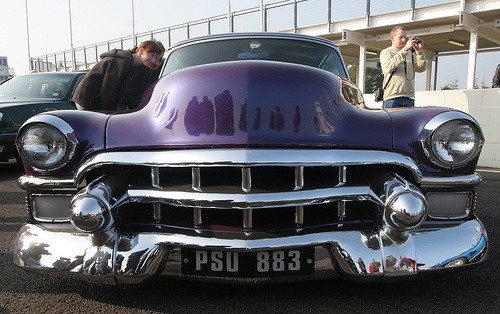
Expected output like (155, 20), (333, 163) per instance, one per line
(0, 71), (88, 166)
(11, 30), (491, 283)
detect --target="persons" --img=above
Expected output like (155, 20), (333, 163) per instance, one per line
(379, 25), (427, 109)
(72, 39), (165, 111)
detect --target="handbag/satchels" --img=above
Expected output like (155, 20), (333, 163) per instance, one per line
(375, 88), (384, 102)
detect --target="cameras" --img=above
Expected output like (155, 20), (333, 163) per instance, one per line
(408, 37), (420, 44)
